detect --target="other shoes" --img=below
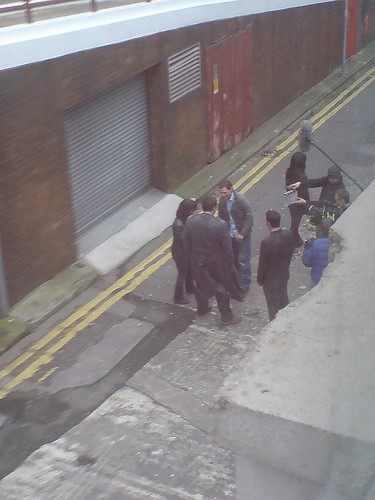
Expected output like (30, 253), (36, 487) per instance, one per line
(197, 307), (211, 315)
(222, 315), (242, 325)
(296, 240), (303, 246)
(174, 298), (190, 304)
(187, 288), (195, 294)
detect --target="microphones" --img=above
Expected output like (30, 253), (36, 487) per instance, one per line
(298, 119), (311, 152)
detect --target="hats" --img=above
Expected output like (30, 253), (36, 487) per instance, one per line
(327, 166), (340, 176)
